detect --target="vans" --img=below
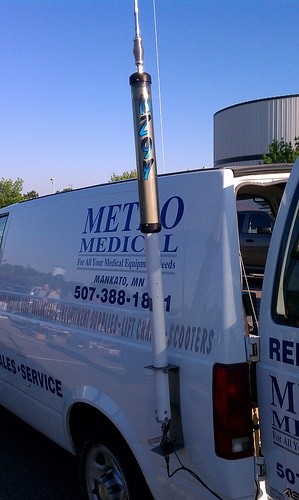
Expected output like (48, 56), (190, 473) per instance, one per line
(0, 159), (299, 500)
